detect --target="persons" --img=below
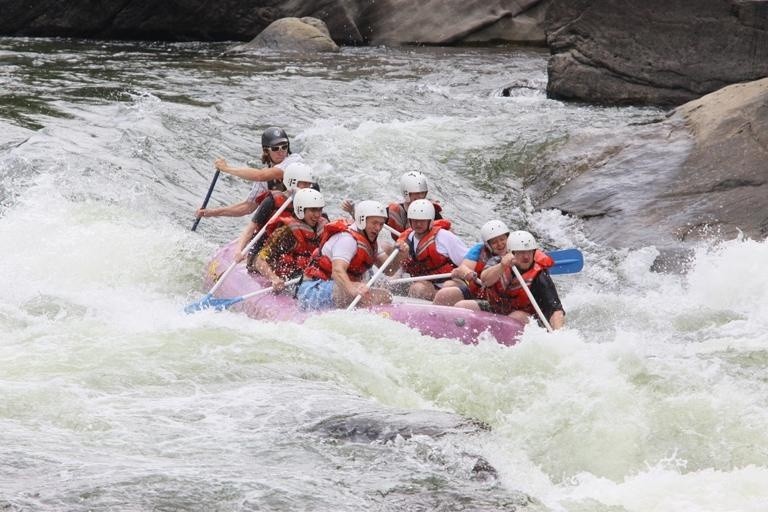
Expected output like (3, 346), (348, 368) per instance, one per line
(296, 199), (409, 312)
(342, 170), (445, 243)
(381, 198), (471, 301)
(454, 229), (566, 333)
(254, 188), (330, 295)
(194, 126), (305, 218)
(234, 161), (319, 273)
(433, 219), (511, 306)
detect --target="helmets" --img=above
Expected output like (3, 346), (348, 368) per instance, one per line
(401, 171), (435, 229)
(262, 127), (289, 148)
(482, 220), (537, 253)
(283, 164), (325, 220)
(355, 201), (388, 231)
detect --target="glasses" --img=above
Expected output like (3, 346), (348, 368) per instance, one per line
(268, 145), (288, 151)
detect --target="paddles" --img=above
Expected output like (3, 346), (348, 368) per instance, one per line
(186, 276), (308, 312)
(392, 248), (584, 283)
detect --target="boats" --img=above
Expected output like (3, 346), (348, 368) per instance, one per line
(202, 233), (527, 350)
